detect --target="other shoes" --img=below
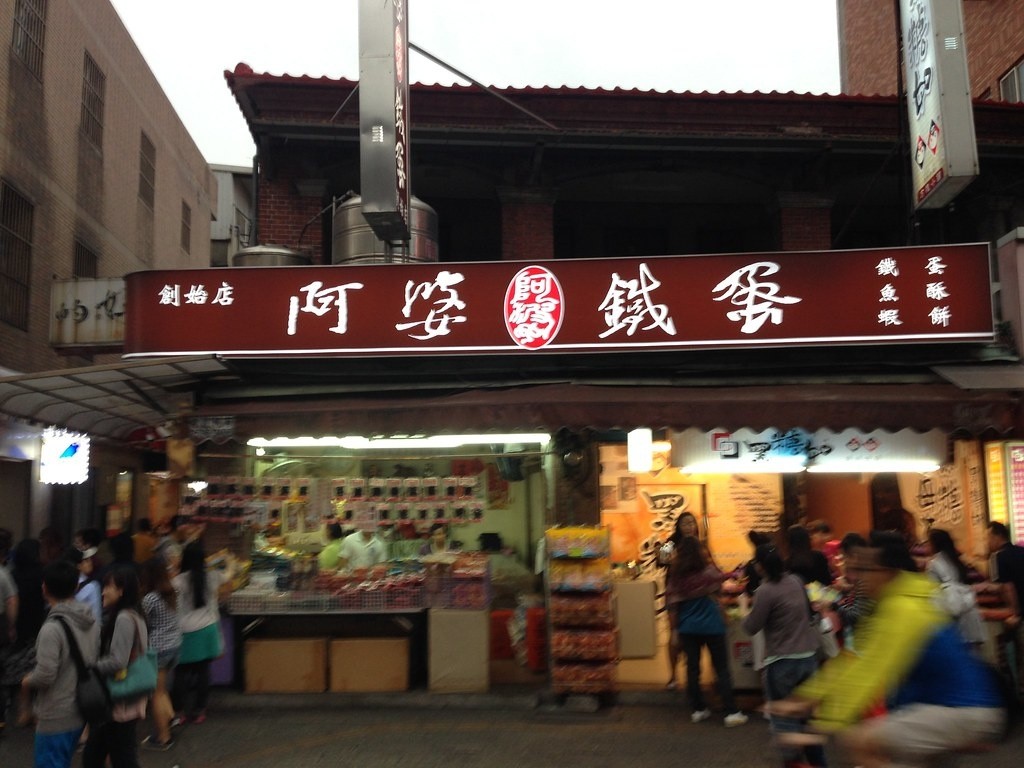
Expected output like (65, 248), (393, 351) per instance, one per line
(723, 710), (749, 729)
(140, 735), (175, 753)
(167, 716), (181, 730)
(692, 707), (713, 725)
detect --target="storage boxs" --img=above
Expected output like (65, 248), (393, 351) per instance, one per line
(230, 569), (491, 611)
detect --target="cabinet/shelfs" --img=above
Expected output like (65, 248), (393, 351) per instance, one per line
(239, 614), (416, 693)
(429, 609), (489, 694)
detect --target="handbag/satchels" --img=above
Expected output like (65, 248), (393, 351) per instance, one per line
(78, 668), (114, 726)
(102, 612), (159, 705)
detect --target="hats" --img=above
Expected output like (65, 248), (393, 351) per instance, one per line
(62, 545), (99, 567)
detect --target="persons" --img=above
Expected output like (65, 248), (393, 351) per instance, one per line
(656, 506), (1024, 768)
(322, 526), (387, 575)
(316, 522), (342, 572)
(479, 534), (535, 611)
(419, 524), (457, 556)
(0, 513), (238, 768)
